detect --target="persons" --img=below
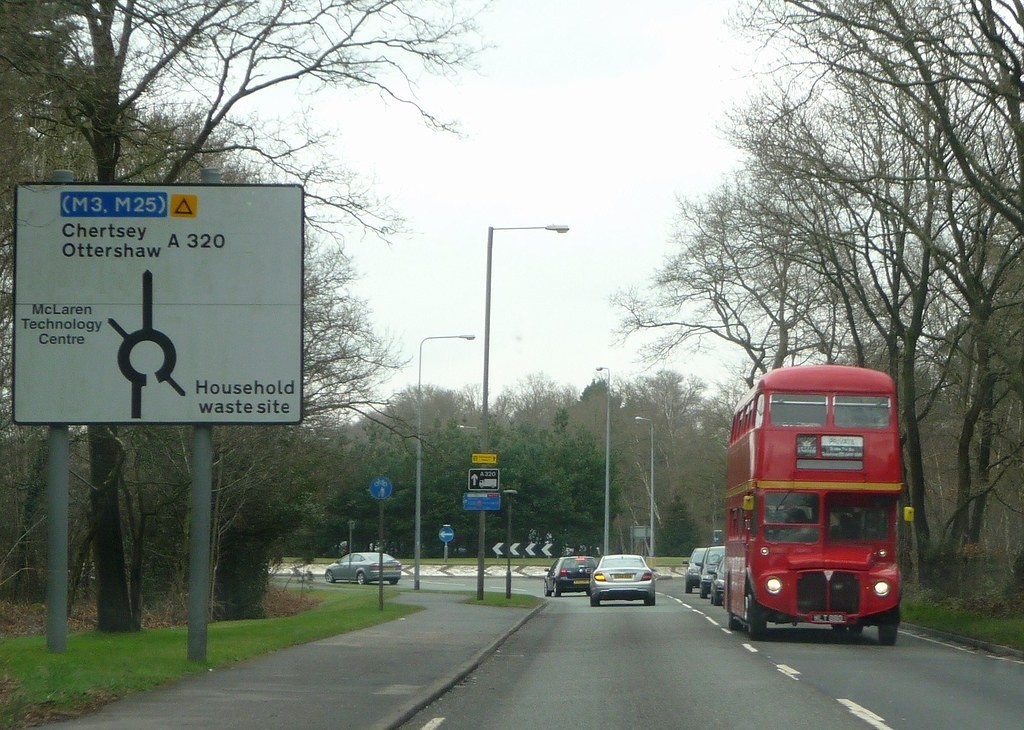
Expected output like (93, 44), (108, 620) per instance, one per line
(830, 509), (885, 540)
(752, 499), (808, 541)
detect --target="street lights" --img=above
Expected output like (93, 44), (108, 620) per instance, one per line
(636, 416), (655, 558)
(474, 223), (570, 602)
(504, 488), (517, 598)
(414, 335), (476, 592)
(595, 367), (611, 557)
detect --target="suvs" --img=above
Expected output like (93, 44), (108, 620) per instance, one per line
(682, 547), (708, 594)
(695, 545), (726, 598)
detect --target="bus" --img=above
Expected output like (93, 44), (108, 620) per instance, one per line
(725, 365), (915, 646)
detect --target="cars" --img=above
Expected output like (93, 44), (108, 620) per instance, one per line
(585, 554), (658, 606)
(325, 552), (403, 586)
(543, 556), (598, 597)
(707, 555), (726, 605)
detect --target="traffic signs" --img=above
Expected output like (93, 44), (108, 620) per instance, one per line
(467, 468), (501, 491)
